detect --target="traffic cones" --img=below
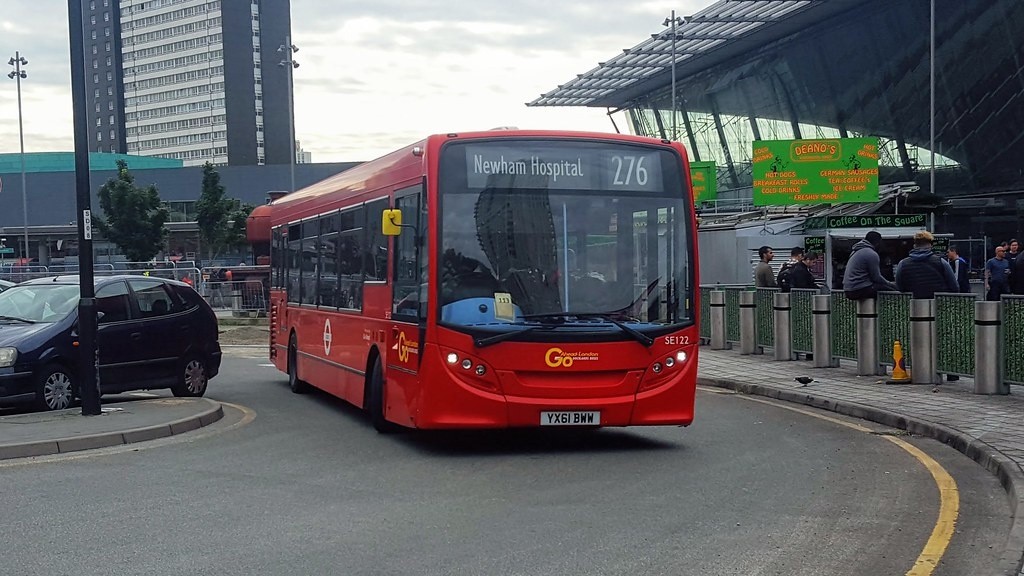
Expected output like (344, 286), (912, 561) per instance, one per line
(886, 340), (912, 385)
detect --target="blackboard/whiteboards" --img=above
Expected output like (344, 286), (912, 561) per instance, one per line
(931, 234), (955, 264)
(804, 236), (826, 281)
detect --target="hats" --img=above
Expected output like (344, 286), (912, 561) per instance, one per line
(865, 231), (881, 243)
(792, 247), (804, 253)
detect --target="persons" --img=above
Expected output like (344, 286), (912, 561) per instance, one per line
(895, 230), (959, 299)
(844, 231), (895, 301)
(754, 246), (777, 288)
(985, 239), (1024, 301)
(945, 244), (971, 293)
(142, 250), (229, 309)
(777, 246), (824, 292)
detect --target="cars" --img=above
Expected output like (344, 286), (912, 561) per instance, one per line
(0, 279), (55, 325)
(0, 275), (223, 413)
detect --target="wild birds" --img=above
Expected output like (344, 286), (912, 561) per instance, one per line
(793, 376), (819, 387)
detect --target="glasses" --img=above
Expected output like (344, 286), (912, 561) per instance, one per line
(998, 250), (1007, 253)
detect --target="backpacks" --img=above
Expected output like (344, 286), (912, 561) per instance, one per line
(776, 262), (798, 290)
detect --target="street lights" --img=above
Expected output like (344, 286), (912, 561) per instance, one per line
(8, 50), (30, 269)
(663, 8), (692, 140)
(278, 34), (300, 195)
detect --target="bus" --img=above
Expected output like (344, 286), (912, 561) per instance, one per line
(247, 130), (704, 434)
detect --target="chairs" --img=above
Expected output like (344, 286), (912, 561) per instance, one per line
(41, 295), (71, 321)
(151, 298), (168, 312)
